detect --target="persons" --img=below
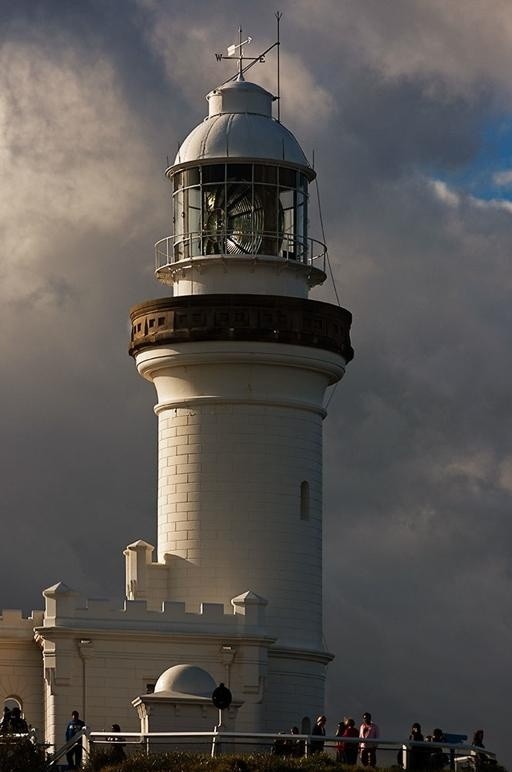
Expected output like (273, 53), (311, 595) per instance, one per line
(424, 728), (442, 741)
(408, 722), (423, 741)
(103, 724), (127, 753)
(0, 705), (31, 734)
(271, 711), (379, 767)
(470, 728), (485, 748)
(64, 710), (85, 767)
